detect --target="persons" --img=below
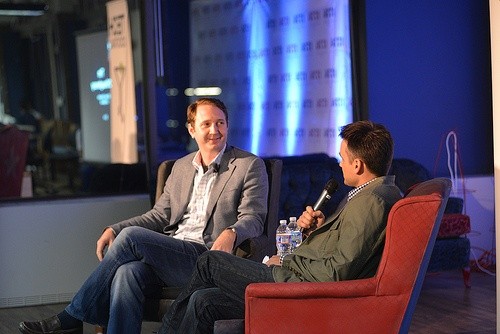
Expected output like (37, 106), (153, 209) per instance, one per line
(159, 120), (404, 334)
(19, 97), (269, 334)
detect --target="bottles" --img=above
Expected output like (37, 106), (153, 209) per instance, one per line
(276, 217), (302, 264)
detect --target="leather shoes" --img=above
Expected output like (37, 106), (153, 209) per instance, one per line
(18, 314), (83, 334)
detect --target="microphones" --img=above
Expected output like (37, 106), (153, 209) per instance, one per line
(299, 178), (339, 233)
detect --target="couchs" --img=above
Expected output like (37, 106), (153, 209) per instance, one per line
(96, 153), (470, 334)
